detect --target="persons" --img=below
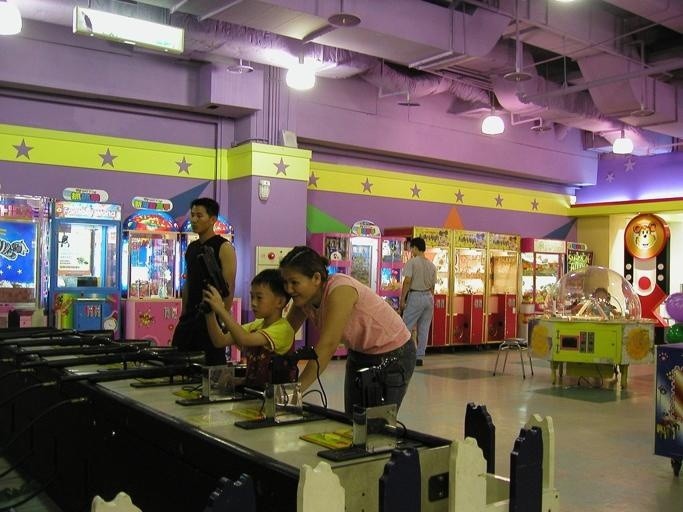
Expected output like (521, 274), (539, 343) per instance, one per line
(400, 237), (437, 366)
(277, 246), (416, 421)
(171, 197), (238, 365)
(199, 268), (298, 390)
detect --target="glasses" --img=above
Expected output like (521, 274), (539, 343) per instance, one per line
(613, 128), (634, 155)
(481, 90), (504, 137)
(285, 55), (316, 93)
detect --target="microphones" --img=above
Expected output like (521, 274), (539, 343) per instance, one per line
(492, 337), (534, 379)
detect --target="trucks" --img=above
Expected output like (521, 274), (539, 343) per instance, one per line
(665, 292), (683, 321)
(666, 322), (683, 345)
